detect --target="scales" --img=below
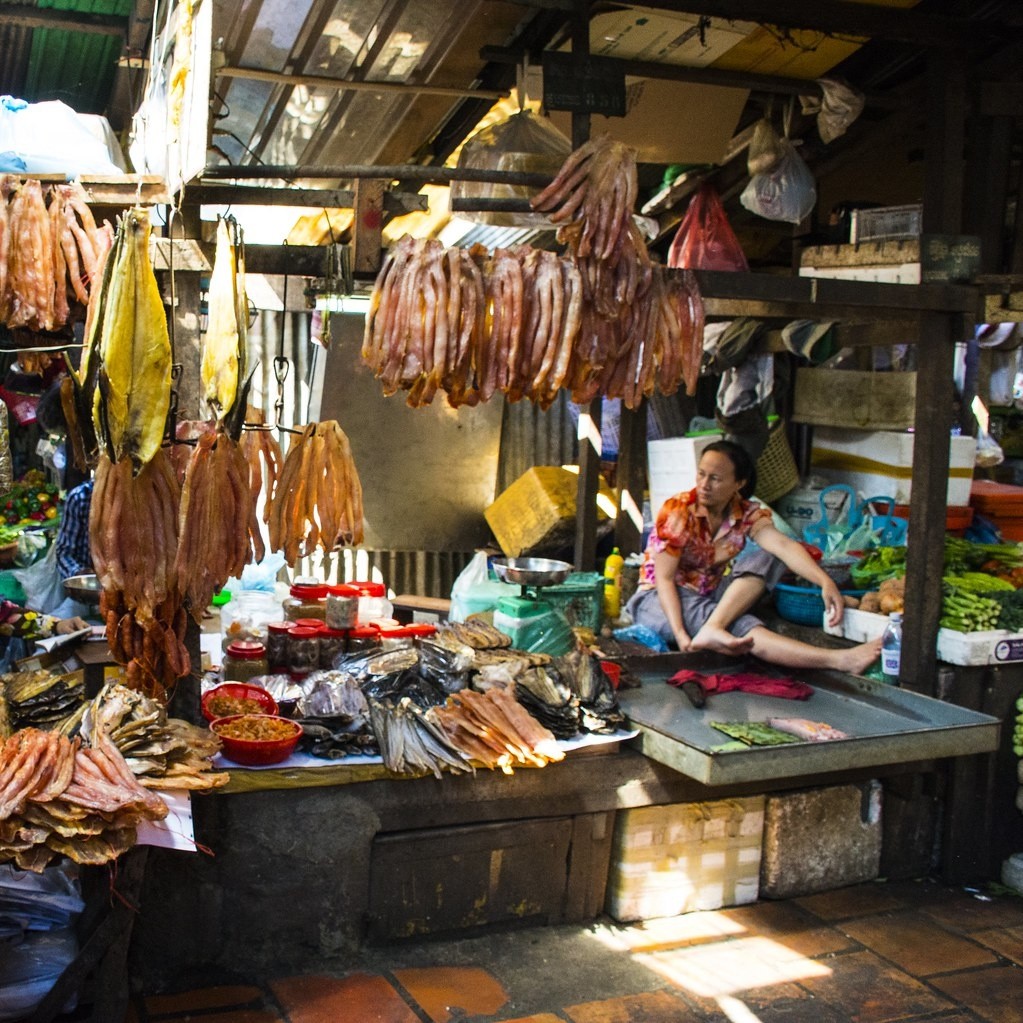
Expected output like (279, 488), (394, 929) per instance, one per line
(489, 555), (581, 659)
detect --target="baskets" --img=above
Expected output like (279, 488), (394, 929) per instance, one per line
(776, 582), (874, 627)
(803, 485), (908, 553)
(210, 714), (303, 766)
(202, 680), (280, 722)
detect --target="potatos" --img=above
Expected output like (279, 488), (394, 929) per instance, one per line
(840, 575), (907, 616)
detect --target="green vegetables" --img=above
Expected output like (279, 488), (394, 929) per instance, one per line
(848, 537), (1023, 632)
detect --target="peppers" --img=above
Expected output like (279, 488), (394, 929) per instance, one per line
(0, 482), (66, 532)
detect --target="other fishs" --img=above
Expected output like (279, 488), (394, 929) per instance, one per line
(0, 134), (704, 873)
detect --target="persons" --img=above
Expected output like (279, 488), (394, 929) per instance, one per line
(626, 441), (884, 674)
(55, 480), (96, 577)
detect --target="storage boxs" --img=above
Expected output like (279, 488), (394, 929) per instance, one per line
(363, 815), (573, 949)
(542, 572), (615, 636)
(822, 605), (1023, 666)
(606, 778), (883, 922)
(10, 649), (85, 700)
(799, 240), (921, 285)
(200, 612), (221, 670)
(483, 465), (618, 560)
(648, 435), (724, 524)
(793, 368), (974, 505)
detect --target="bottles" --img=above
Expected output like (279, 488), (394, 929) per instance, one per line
(223, 582), (436, 684)
(881, 613), (903, 685)
(604, 547), (624, 617)
